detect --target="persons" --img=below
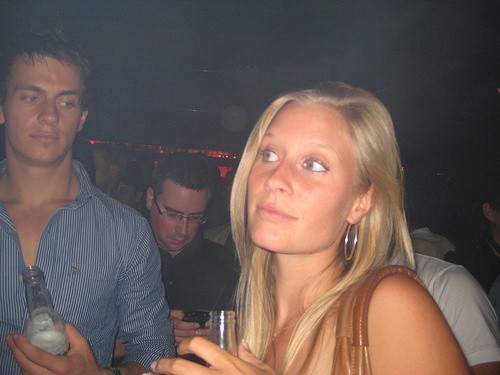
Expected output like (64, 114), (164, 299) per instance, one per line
(89, 145), (133, 207)
(111, 150), (242, 367)
(141, 77), (475, 375)
(130, 147), (239, 249)
(385, 179), (500, 375)
(1, 24), (177, 374)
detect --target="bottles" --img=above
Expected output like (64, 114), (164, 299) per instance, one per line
(21, 266), (71, 356)
(200, 310), (240, 369)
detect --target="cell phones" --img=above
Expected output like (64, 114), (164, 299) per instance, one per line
(181, 311), (211, 327)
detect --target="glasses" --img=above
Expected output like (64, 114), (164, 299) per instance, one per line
(152, 187), (205, 223)
